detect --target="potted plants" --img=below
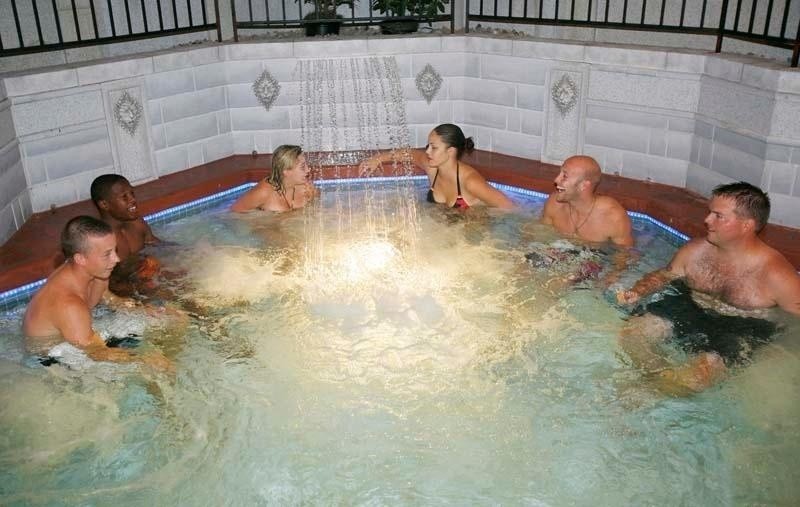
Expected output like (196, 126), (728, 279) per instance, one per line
(292, 0), (358, 39)
(369, 0), (453, 35)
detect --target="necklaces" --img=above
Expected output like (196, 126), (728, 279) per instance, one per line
(280, 187), (295, 211)
(570, 197), (596, 239)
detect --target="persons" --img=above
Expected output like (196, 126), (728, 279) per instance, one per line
(358, 124), (513, 219)
(231, 145), (321, 214)
(91, 174), (203, 316)
(522, 155), (633, 293)
(23, 215), (184, 395)
(616, 182), (800, 402)
